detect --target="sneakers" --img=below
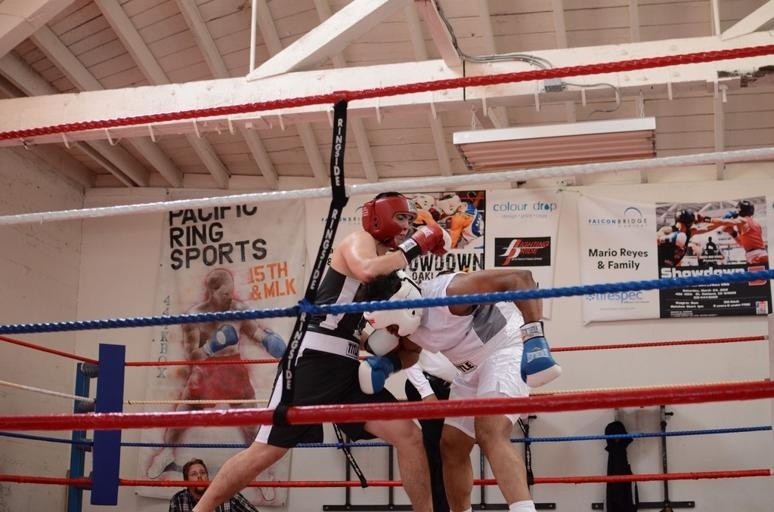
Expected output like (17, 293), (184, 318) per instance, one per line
(148, 452), (175, 479)
(256, 469), (274, 501)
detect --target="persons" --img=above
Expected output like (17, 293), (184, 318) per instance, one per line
(358, 269), (559, 511)
(189, 193), (453, 512)
(144, 268), (287, 501)
(657, 206), (740, 274)
(693, 200), (770, 282)
(406, 192), (485, 252)
(168, 458), (259, 512)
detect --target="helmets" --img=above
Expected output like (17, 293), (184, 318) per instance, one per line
(436, 195), (461, 216)
(412, 194), (434, 211)
(362, 197), (410, 249)
(362, 270), (424, 337)
(675, 208), (695, 227)
(736, 199), (754, 217)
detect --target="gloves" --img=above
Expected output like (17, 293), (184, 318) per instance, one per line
(722, 225), (737, 238)
(694, 213), (712, 224)
(398, 223), (452, 265)
(252, 327), (286, 358)
(519, 321), (563, 388)
(720, 209), (738, 219)
(202, 324), (239, 357)
(660, 231), (687, 249)
(359, 354), (402, 394)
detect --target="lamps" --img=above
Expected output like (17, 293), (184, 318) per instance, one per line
(453, 90), (657, 172)
